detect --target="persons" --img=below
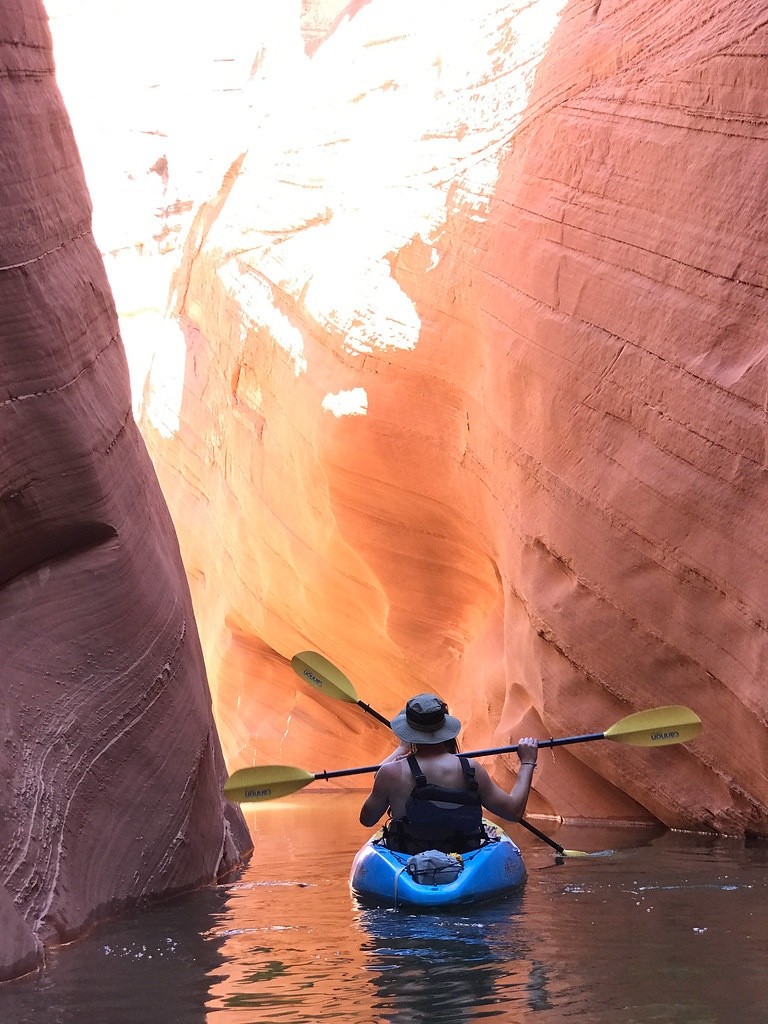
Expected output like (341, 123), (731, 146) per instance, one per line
(359, 693), (539, 854)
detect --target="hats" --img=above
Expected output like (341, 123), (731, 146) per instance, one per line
(390, 694), (461, 744)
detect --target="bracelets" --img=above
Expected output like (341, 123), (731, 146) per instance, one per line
(520, 761), (537, 767)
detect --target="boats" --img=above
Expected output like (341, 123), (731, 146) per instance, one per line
(346, 813), (530, 909)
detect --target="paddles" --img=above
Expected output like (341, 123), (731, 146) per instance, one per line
(224, 703), (703, 803)
(288, 650), (562, 852)
